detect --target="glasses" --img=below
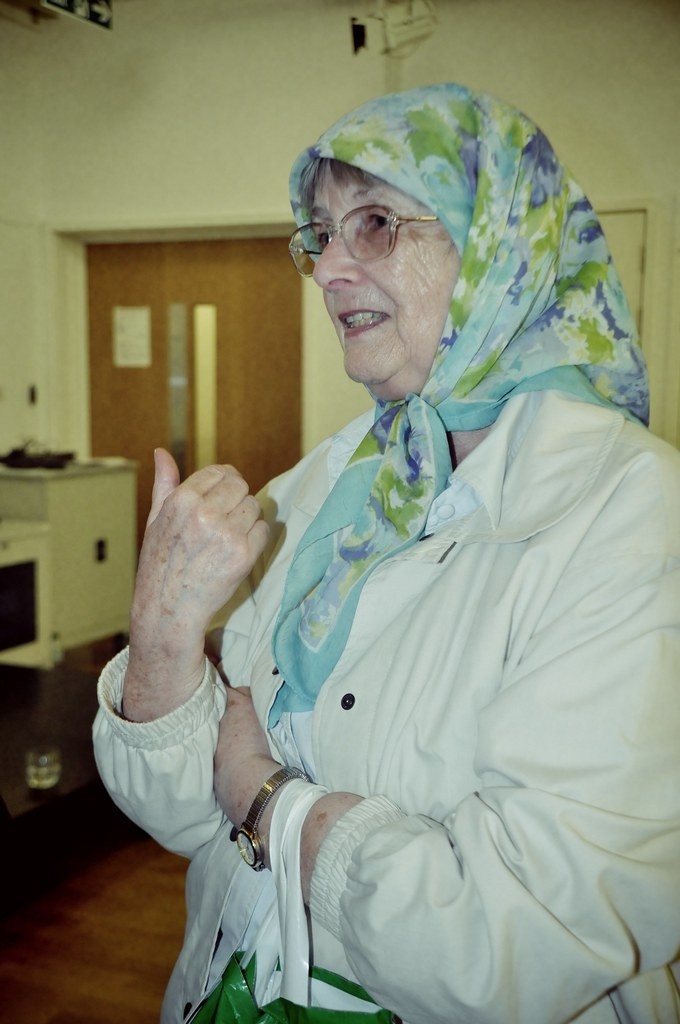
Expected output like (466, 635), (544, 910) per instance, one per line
(288, 204), (439, 278)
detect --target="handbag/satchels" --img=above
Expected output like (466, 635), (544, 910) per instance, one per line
(180, 779), (403, 1024)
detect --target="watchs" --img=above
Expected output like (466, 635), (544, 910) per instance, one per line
(237, 767), (310, 871)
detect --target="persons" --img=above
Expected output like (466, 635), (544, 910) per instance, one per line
(91, 82), (680, 1024)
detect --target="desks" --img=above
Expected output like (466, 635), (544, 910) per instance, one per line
(0, 662), (98, 818)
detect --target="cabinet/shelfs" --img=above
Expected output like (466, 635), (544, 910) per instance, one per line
(0, 457), (139, 654)
